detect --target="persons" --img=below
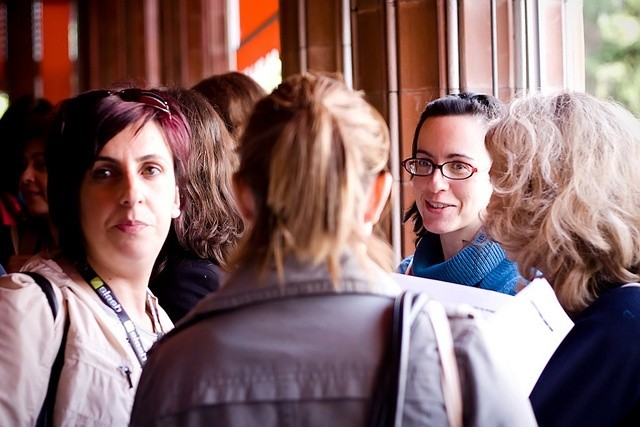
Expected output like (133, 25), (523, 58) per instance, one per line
(124, 73), (542, 427)
(484, 91), (640, 427)
(0, 73), (543, 333)
(0, 88), (190, 427)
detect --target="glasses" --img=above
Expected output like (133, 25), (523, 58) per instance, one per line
(401, 157), (478, 180)
(77, 88), (170, 112)
